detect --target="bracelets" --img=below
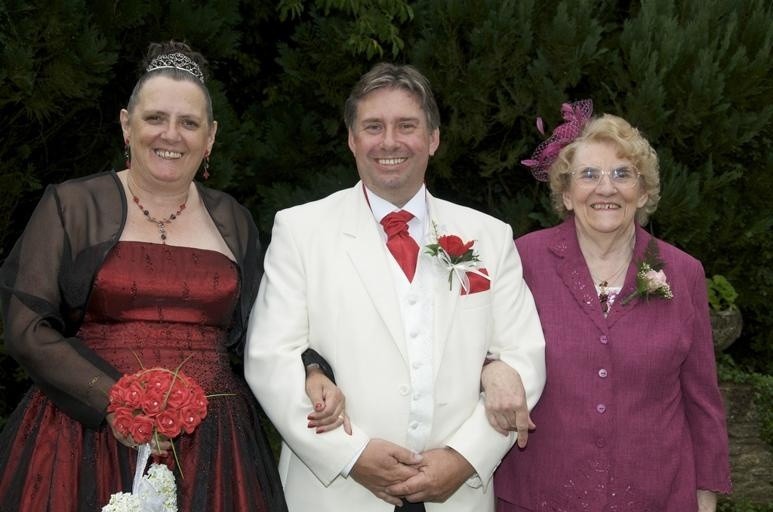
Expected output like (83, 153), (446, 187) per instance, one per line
(84, 371), (105, 407)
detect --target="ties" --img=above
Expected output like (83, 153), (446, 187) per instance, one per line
(381, 210), (421, 284)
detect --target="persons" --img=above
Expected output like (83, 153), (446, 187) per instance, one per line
(241, 60), (548, 510)
(470, 115), (734, 511)
(1, 36), (288, 510)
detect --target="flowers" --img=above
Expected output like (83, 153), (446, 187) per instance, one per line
(423, 218), (495, 293)
(103, 345), (236, 475)
(619, 237), (674, 309)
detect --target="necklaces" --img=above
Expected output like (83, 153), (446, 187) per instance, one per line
(123, 175), (190, 246)
(577, 238), (634, 313)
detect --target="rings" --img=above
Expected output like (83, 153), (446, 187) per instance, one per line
(337, 415), (343, 420)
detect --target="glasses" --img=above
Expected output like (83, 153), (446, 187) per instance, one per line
(570, 167), (640, 188)
